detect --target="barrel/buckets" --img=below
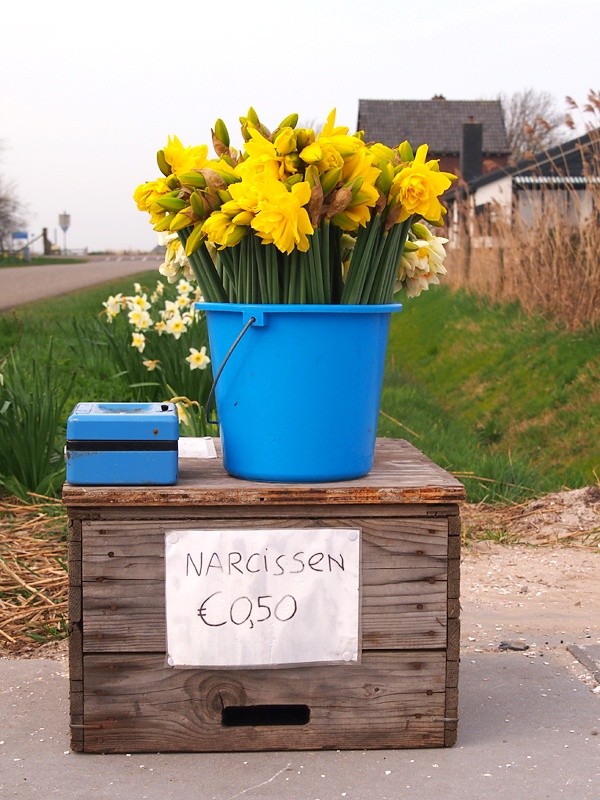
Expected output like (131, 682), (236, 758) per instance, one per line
(195, 300), (405, 487)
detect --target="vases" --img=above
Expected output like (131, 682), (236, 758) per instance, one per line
(192, 296), (404, 488)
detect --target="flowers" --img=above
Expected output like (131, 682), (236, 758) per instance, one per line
(100, 243), (218, 439)
(133, 106), (460, 311)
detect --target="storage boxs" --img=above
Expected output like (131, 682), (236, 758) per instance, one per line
(57, 425), (469, 754)
(64, 400), (179, 486)
